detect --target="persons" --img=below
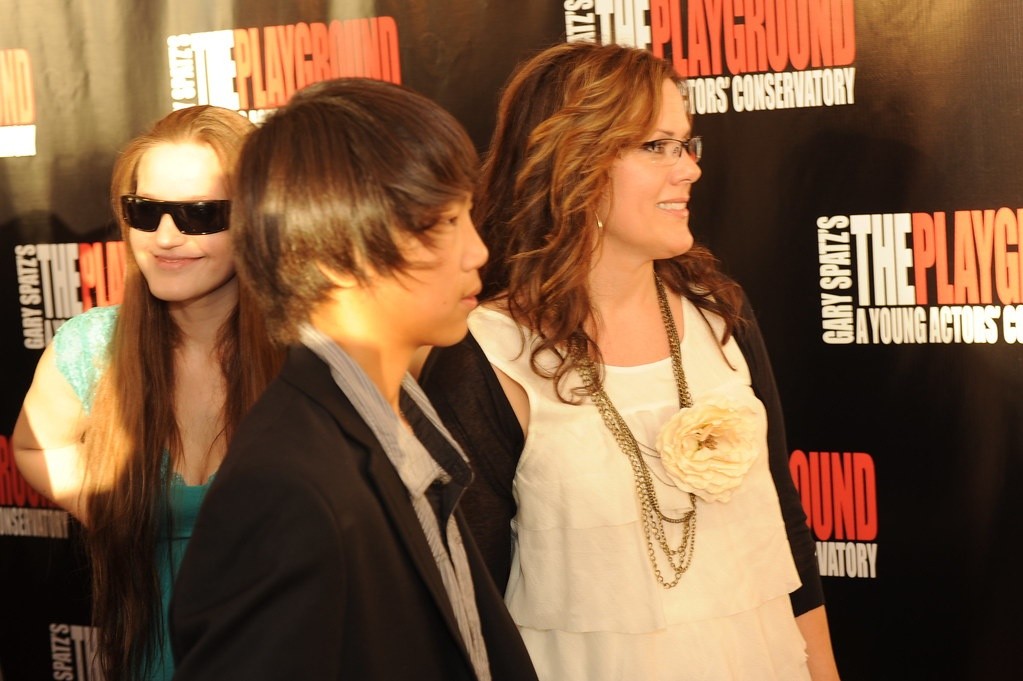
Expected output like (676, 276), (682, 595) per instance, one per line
(466, 43), (839, 680)
(10, 107), (261, 679)
(168, 79), (539, 678)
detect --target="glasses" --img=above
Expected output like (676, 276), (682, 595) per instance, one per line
(119, 194), (230, 235)
(631, 135), (702, 166)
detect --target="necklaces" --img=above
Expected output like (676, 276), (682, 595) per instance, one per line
(537, 263), (698, 590)
